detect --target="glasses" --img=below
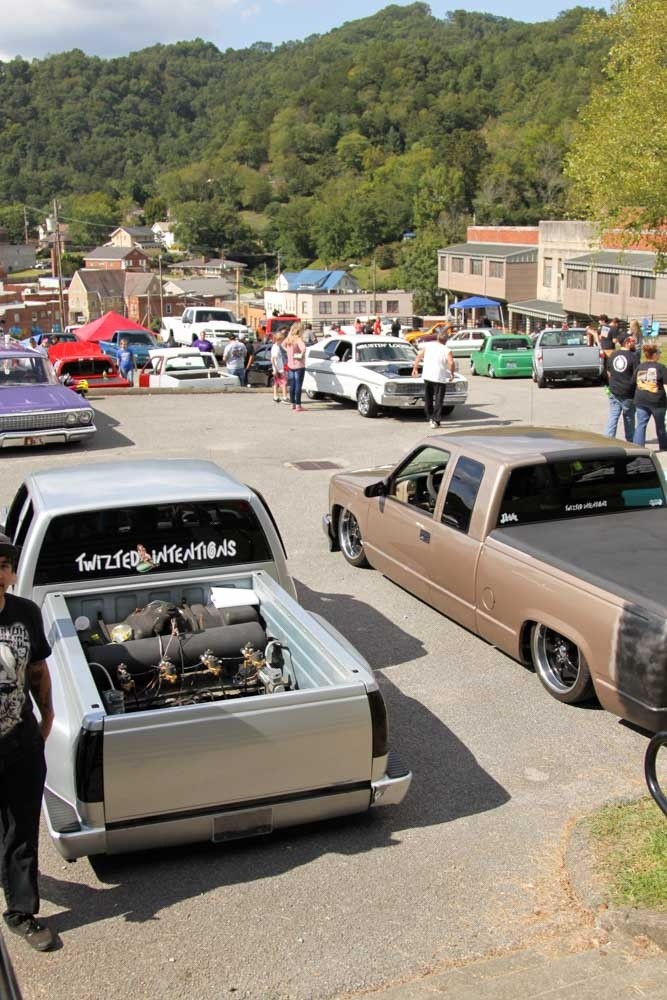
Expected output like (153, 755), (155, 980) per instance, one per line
(655, 351), (662, 355)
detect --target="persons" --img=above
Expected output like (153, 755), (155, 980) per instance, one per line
(281, 322), (308, 411)
(479, 316), (491, 327)
(40, 338), (49, 350)
(238, 334), (255, 371)
(117, 339), (137, 378)
(354, 317), (382, 335)
(272, 333), (288, 404)
(303, 324), (317, 346)
(0, 532), (57, 952)
(330, 326), (344, 336)
(530, 321), (558, 346)
(25, 338), (48, 369)
(410, 335), (455, 428)
(193, 331), (214, 366)
(391, 319), (401, 337)
(596, 314), (667, 452)
(50, 335), (59, 344)
(223, 334), (246, 387)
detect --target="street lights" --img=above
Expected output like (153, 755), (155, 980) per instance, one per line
(348, 258), (377, 315)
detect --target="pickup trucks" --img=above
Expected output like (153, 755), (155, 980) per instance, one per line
(1, 459), (414, 866)
(159, 307), (254, 357)
(321, 425), (667, 741)
(46, 342), (131, 389)
(531, 327), (605, 389)
(98, 330), (160, 368)
(128, 347), (242, 388)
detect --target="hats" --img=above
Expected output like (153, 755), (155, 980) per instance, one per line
(0, 526), (20, 562)
(617, 332), (633, 344)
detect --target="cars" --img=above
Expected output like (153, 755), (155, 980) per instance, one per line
(0, 341), (97, 451)
(245, 344), (274, 387)
(255, 315), (302, 344)
(322, 316), (482, 345)
(301, 333), (470, 419)
(470, 334), (534, 379)
(33, 324), (84, 345)
(445, 328), (504, 357)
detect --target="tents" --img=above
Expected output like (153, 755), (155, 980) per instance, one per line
(450, 296), (505, 334)
(71, 311), (158, 343)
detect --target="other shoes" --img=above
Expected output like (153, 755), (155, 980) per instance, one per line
(272, 398), (282, 403)
(282, 398), (290, 404)
(430, 419), (443, 428)
(296, 406), (308, 411)
(291, 404), (296, 409)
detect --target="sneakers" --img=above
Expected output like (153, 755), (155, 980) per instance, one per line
(8, 916), (56, 951)
